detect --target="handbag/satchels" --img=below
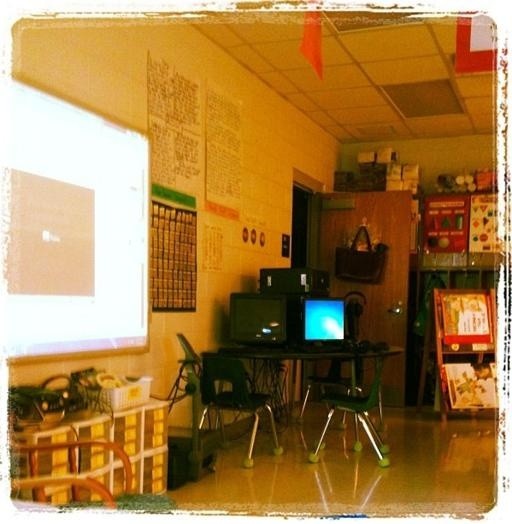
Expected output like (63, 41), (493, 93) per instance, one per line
(335, 227), (386, 283)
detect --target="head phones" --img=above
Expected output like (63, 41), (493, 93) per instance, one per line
(343, 290), (368, 317)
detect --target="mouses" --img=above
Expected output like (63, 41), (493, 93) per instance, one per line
(376, 341), (389, 350)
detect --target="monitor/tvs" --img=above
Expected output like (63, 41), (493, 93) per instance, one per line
(229, 293), (287, 353)
(348, 298), (360, 341)
(300, 296), (347, 343)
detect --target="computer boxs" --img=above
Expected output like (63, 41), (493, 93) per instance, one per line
(259, 267), (329, 294)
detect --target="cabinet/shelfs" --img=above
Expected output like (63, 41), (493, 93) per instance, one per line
(9, 399), (172, 507)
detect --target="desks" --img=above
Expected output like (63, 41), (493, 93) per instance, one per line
(215, 343), (406, 451)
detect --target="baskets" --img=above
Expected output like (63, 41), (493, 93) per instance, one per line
(77, 376), (152, 411)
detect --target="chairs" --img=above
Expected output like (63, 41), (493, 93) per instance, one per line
(298, 339), (392, 470)
(199, 353), (286, 469)
